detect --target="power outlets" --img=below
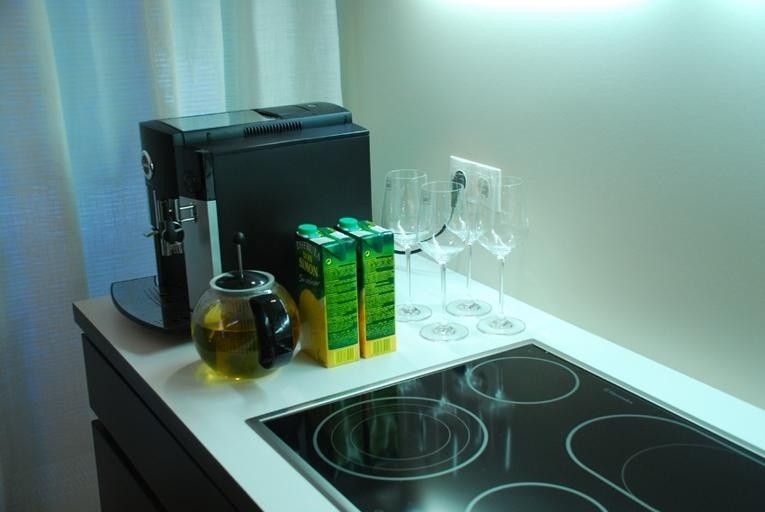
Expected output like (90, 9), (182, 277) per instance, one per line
(450, 157), (502, 213)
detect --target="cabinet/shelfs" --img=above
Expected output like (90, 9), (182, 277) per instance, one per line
(72, 247), (765, 512)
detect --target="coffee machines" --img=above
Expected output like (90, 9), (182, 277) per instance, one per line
(110, 101), (374, 338)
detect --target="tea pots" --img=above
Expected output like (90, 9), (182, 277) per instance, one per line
(191, 230), (301, 381)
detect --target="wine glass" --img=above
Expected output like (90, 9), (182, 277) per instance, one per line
(415, 182), (469, 342)
(473, 177), (529, 335)
(441, 169), (493, 317)
(381, 170), (430, 322)
